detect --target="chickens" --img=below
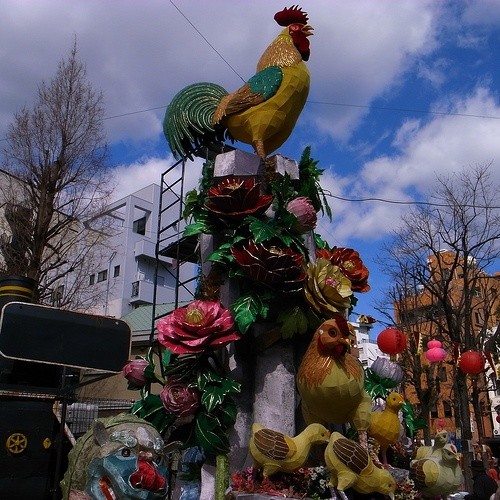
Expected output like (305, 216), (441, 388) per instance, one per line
(408, 443), (463, 500)
(368, 393), (406, 464)
(324, 429), (396, 500)
(296, 315), (372, 454)
(162, 4), (314, 166)
(249, 421), (330, 479)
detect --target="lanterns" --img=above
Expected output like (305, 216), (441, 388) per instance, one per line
(460, 352), (484, 374)
(376, 328), (406, 355)
(372, 357), (405, 391)
(428, 340), (443, 349)
(425, 347), (448, 364)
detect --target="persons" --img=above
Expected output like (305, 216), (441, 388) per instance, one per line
(463, 460), (499, 500)
